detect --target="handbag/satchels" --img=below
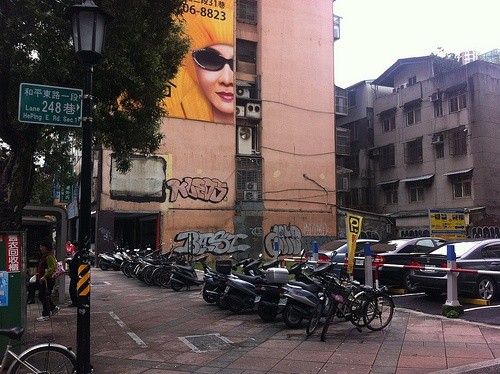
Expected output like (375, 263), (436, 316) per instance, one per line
(50, 265), (65, 278)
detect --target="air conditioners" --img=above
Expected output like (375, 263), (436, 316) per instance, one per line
(430, 94), (440, 102)
(237, 106), (244, 117)
(432, 136), (443, 144)
(246, 103), (261, 118)
(243, 191), (258, 201)
(236, 86), (250, 98)
(246, 182), (258, 190)
(337, 178), (348, 190)
(392, 83), (409, 92)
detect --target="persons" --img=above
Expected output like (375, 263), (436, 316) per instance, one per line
(65, 240), (75, 258)
(65, 240), (78, 307)
(171, 0), (235, 125)
(35, 240), (60, 322)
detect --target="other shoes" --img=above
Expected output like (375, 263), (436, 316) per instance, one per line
(68, 304), (76, 307)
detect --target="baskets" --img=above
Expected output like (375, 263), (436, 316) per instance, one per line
(327, 279), (351, 303)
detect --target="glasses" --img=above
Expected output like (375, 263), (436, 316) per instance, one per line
(192, 49), (234, 72)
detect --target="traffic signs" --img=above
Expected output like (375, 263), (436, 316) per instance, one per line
(17, 81), (84, 127)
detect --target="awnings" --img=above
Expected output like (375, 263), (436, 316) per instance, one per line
(376, 180), (399, 187)
(442, 168), (473, 183)
(400, 174), (434, 185)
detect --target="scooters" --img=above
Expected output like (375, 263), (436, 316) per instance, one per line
(89, 239), (396, 342)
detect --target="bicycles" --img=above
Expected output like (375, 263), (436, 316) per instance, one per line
(0, 325), (77, 374)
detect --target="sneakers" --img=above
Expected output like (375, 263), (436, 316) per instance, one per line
(52, 306), (60, 316)
(37, 316), (49, 320)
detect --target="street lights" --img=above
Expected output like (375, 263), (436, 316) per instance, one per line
(66, 0), (115, 374)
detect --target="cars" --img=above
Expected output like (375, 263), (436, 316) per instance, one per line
(408, 237), (500, 303)
(304, 238), (448, 294)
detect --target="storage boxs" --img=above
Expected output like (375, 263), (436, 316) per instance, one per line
(266, 268), (289, 283)
(216, 259), (232, 274)
